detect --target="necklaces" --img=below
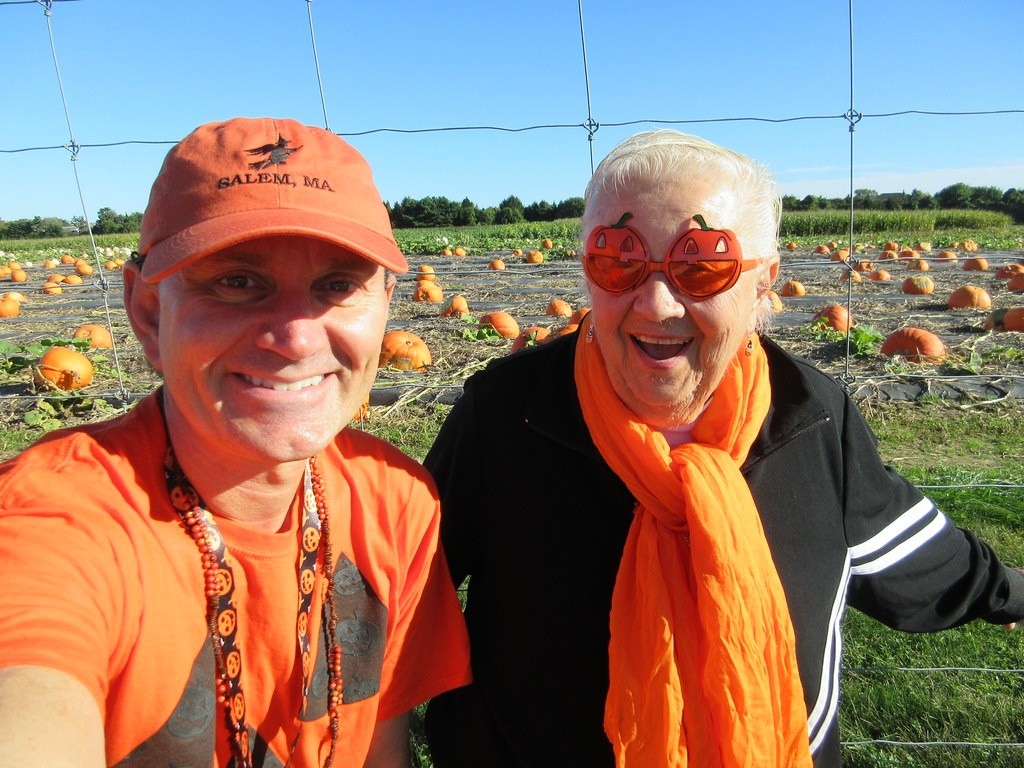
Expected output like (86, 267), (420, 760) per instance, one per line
(156, 385), (344, 768)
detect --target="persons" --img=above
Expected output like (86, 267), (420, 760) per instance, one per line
(0, 119), (470, 768)
(419, 129), (1023, 768)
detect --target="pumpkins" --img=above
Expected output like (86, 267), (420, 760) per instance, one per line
(766, 240), (1024, 366)
(0, 246), (131, 390)
(350, 238), (592, 415)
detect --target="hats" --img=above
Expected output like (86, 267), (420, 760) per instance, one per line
(138, 117), (408, 284)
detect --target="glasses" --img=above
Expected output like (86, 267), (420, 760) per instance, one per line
(580, 212), (767, 301)
